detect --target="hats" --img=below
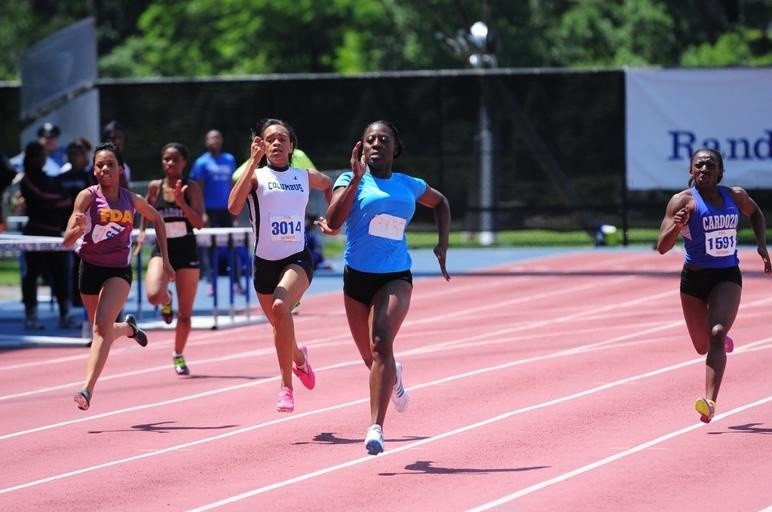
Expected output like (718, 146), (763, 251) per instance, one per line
(38, 122), (61, 136)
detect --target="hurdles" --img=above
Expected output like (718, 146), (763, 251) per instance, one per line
(0, 228), (269, 348)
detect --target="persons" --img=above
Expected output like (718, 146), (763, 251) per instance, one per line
(227, 117), (333, 414)
(658, 147), (772, 423)
(51, 136), (91, 309)
(60, 136), (94, 174)
(133, 142), (204, 376)
(8, 122), (62, 176)
(19, 142), (82, 328)
(229, 147), (317, 317)
(85, 120), (129, 348)
(325, 119), (452, 454)
(62, 143), (177, 410)
(189, 129), (237, 297)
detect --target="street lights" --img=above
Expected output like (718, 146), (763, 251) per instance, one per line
(464, 19), (503, 244)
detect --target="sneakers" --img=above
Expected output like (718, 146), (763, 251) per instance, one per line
(125, 314), (148, 347)
(172, 350), (189, 374)
(275, 345), (315, 412)
(365, 424), (384, 454)
(161, 289), (173, 323)
(723, 335), (733, 352)
(694, 397), (715, 423)
(74, 389), (89, 410)
(391, 362), (409, 413)
